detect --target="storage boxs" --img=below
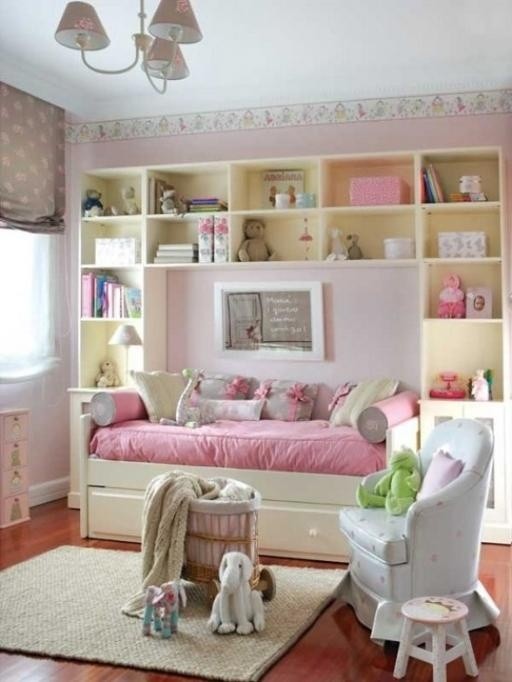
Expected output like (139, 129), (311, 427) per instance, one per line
(349, 177), (409, 205)
(438, 231), (486, 259)
(96, 238), (141, 266)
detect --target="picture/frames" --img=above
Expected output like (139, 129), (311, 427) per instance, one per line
(213, 281), (322, 361)
(465, 285), (491, 319)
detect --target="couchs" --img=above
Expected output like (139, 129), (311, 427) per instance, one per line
(71, 370), (421, 565)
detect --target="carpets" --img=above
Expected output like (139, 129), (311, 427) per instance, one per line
(2, 544), (352, 681)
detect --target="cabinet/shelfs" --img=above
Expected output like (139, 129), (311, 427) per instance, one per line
(2, 409), (32, 526)
(67, 145), (510, 548)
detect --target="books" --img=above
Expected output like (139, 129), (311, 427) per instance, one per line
(80, 171), (144, 321)
(151, 179), (230, 263)
(423, 163), (444, 204)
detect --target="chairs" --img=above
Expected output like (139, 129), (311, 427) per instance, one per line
(340, 418), (500, 637)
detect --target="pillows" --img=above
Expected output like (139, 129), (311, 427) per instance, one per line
(418, 449), (462, 499)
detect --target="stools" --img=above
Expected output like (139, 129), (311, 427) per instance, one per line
(392, 594), (479, 681)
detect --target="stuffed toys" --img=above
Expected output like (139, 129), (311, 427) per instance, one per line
(355, 444), (420, 515)
(139, 580), (188, 638)
(207, 550), (266, 635)
(434, 271), (466, 318)
(236, 218), (279, 262)
(344, 234), (365, 261)
(323, 225), (349, 262)
(95, 359), (122, 387)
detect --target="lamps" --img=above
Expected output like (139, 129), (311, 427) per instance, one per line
(106, 320), (144, 386)
(52, 2), (203, 96)
